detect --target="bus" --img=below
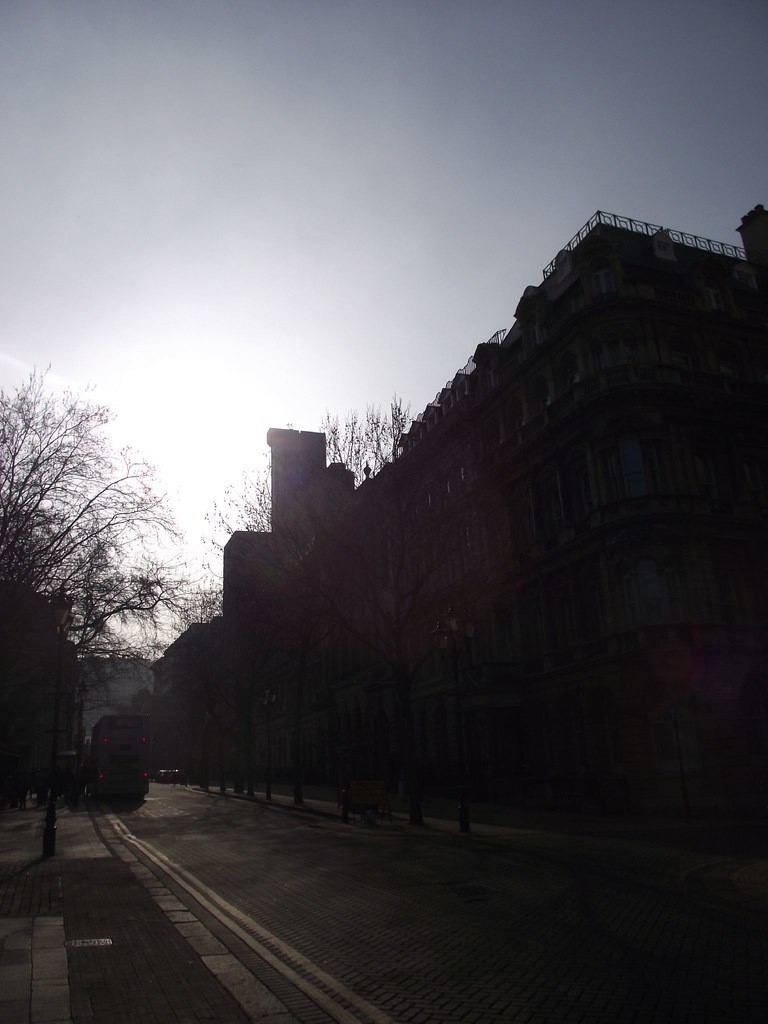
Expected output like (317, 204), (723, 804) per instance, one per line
(90, 712), (152, 801)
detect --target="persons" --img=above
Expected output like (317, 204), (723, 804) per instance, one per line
(1, 763), (95, 812)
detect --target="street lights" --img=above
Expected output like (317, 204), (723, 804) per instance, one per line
(429, 605), (480, 833)
(38, 586), (75, 856)
(259, 688), (279, 802)
